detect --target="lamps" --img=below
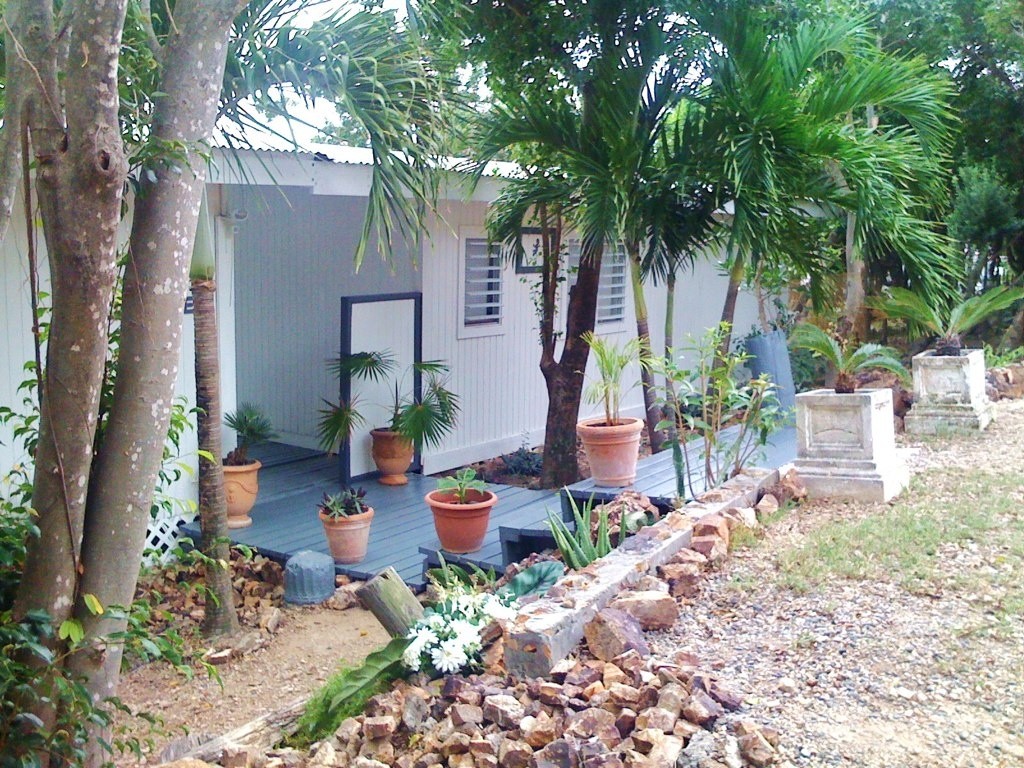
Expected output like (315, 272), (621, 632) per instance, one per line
(230, 207), (249, 222)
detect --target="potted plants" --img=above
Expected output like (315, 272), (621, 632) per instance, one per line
(317, 486), (375, 564)
(864, 285), (1024, 435)
(423, 466), (497, 552)
(221, 401), (280, 530)
(573, 330), (663, 488)
(316, 351), (465, 485)
(785, 320), (912, 499)
